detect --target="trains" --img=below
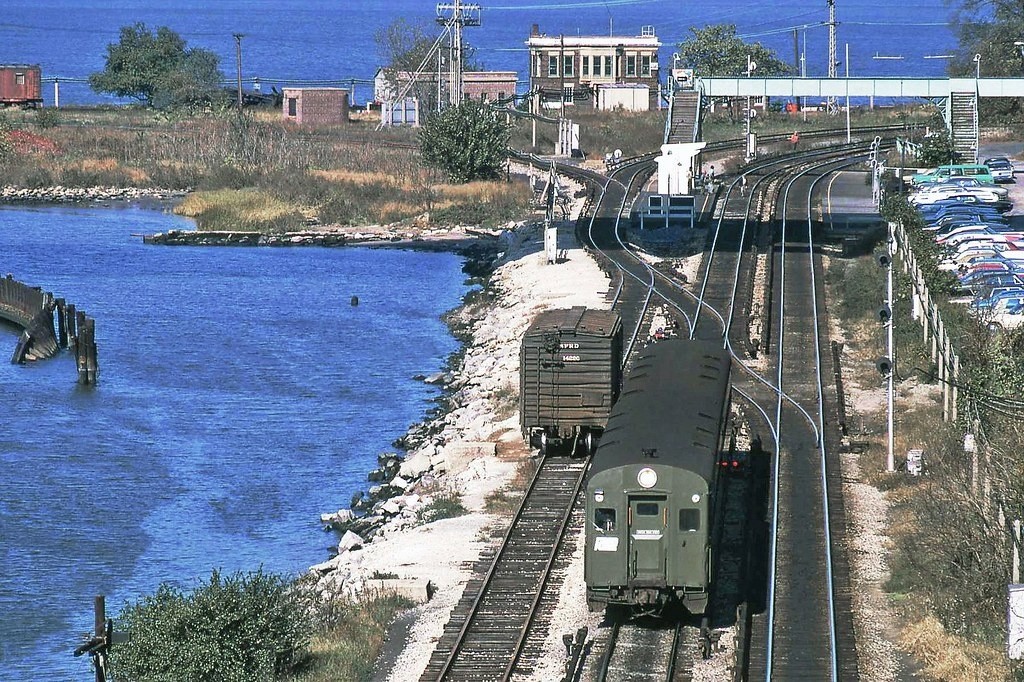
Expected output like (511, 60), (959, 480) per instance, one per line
(582, 338), (732, 620)
(518, 304), (622, 457)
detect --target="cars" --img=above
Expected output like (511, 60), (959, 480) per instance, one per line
(905, 163), (1024, 335)
(984, 158), (1014, 182)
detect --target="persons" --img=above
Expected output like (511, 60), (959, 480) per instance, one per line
(791, 131), (798, 150)
